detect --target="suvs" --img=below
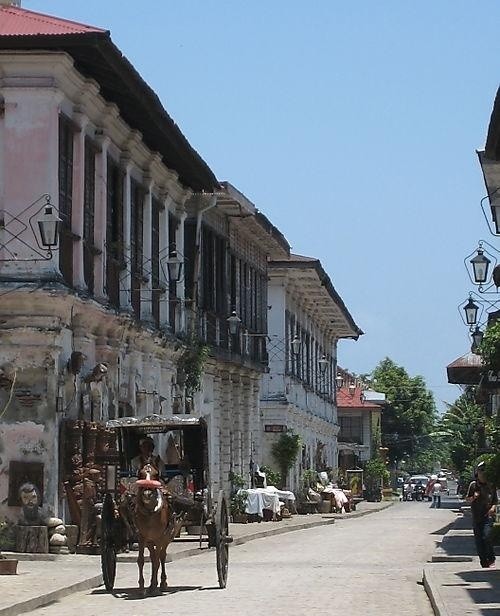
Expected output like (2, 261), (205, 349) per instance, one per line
(404, 477), (430, 499)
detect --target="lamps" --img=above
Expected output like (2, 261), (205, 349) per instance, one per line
(335, 373), (344, 389)
(0, 194), (68, 262)
(360, 391), (366, 404)
(480, 187), (500, 236)
(458, 290), (500, 326)
(118, 241), (190, 293)
(348, 382), (356, 398)
(227, 311), (241, 335)
(290, 334), (302, 356)
(467, 324), (485, 347)
(318, 355), (329, 373)
(464, 240), (500, 293)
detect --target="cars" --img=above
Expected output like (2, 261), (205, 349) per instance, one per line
(427, 473), (448, 491)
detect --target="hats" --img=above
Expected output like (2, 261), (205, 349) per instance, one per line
(140, 438), (155, 445)
(477, 461), (487, 472)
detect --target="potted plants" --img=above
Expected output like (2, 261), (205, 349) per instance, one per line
(229, 492), (250, 523)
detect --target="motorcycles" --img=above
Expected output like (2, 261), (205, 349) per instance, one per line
(406, 487), (437, 508)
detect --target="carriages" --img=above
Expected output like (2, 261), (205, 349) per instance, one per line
(102, 415), (232, 592)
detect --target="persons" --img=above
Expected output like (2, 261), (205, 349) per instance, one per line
(127, 436), (171, 480)
(465, 461), (498, 569)
(15, 481), (50, 525)
(402, 478), (444, 503)
(430, 479), (443, 508)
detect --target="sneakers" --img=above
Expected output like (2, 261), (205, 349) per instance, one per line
(488, 560), (496, 569)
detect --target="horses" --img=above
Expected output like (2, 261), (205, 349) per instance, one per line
(128, 454), (176, 597)
